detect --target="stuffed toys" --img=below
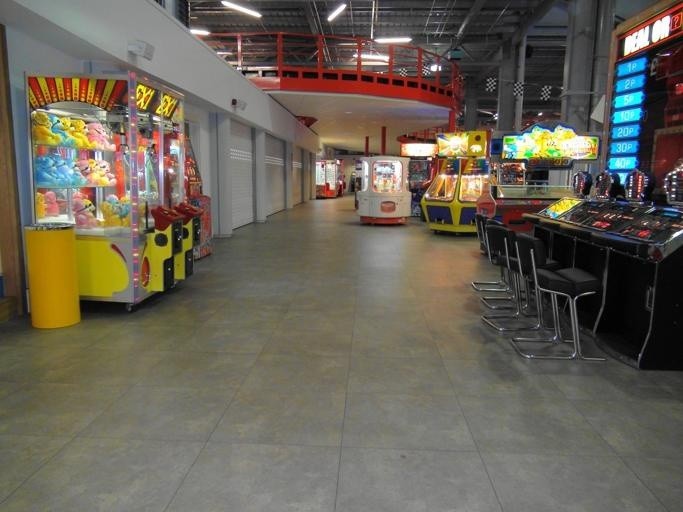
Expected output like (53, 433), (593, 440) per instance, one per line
(30, 108), (147, 229)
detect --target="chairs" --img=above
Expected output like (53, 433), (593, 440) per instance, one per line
(469, 214), (605, 360)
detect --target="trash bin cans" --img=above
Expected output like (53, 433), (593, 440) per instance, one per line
(24, 222), (81, 329)
